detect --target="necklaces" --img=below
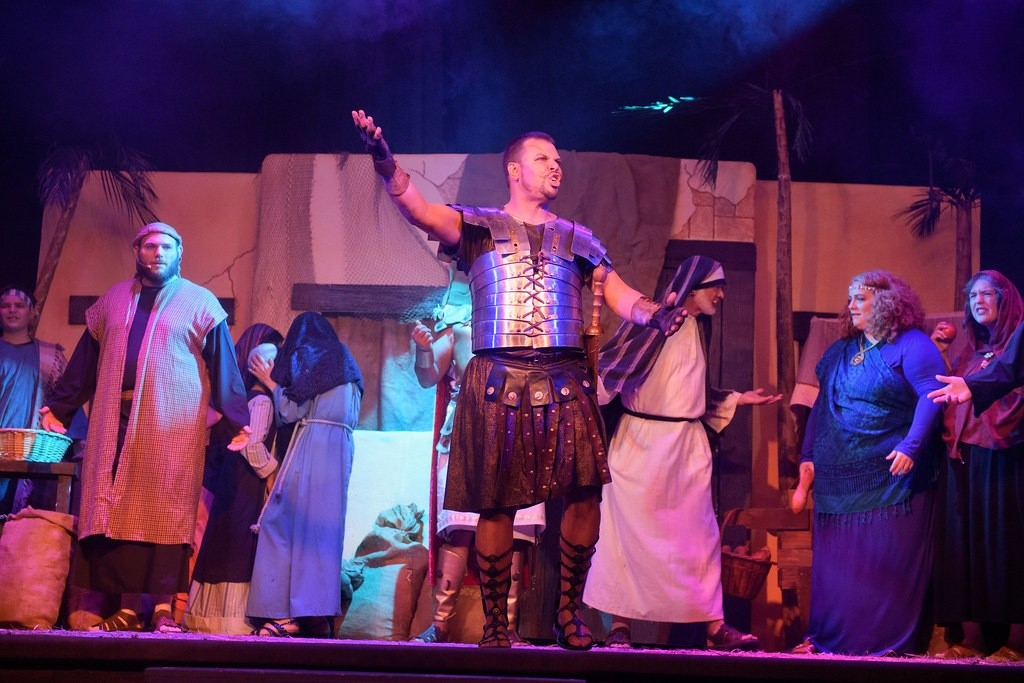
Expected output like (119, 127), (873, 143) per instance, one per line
(849, 334), (879, 366)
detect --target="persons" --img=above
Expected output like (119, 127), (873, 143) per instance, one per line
(181, 322), (296, 633)
(39, 222), (253, 633)
(0, 281), (87, 630)
(597, 254), (783, 650)
(248, 311), (364, 637)
(408, 303), (546, 646)
(792, 271), (950, 658)
(930, 270), (1024, 663)
(927, 320), (1024, 418)
(352, 110), (688, 651)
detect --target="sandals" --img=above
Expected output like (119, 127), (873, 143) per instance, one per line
(412, 625), (453, 643)
(706, 624), (758, 649)
(249, 619), (301, 637)
(93, 611), (147, 632)
(935, 646), (986, 658)
(605, 627), (631, 648)
(983, 646), (1024, 661)
(151, 609), (181, 633)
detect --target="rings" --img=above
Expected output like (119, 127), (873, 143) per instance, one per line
(253, 365), (256, 371)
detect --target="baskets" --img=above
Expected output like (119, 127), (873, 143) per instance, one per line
(0, 428), (73, 463)
(719, 507), (772, 602)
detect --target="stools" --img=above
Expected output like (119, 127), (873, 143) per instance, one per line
(0, 460), (81, 515)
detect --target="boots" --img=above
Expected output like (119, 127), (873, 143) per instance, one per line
(552, 530), (600, 650)
(473, 546), (513, 647)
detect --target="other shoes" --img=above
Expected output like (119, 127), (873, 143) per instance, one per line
(793, 641), (817, 654)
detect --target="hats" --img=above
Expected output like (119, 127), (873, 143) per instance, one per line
(132, 222), (182, 246)
